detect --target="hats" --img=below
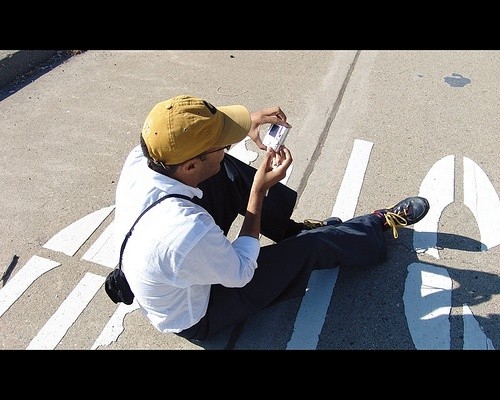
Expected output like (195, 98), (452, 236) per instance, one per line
(142, 95), (252, 166)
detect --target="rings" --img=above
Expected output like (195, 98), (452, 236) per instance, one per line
(277, 163), (281, 166)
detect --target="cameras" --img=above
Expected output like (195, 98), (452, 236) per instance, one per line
(262, 123), (289, 153)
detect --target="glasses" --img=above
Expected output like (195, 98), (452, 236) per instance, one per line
(175, 144), (231, 169)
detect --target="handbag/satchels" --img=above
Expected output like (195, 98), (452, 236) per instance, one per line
(105, 269), (135, 305)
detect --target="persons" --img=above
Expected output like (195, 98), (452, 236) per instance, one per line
(114, 95), (430, 343)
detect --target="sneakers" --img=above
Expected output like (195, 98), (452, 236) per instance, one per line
(372, 197), (430, 233)
(304, 217), (342, 230)
